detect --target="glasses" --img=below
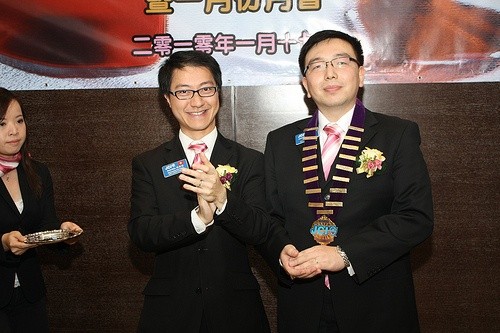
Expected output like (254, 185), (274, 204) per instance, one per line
(304, 57), (360, 76)
(169, 85), (218, 100)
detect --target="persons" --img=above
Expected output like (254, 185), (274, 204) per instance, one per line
(0, 87), (84, 333)
(128, 46), (270, 333)
(262, 30), (434, 333)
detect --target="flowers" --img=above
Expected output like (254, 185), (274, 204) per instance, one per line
(356, 147), (386, 178)
(214, 164), (238, 191)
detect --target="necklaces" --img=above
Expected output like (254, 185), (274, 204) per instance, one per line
(6, 175), (9, 181)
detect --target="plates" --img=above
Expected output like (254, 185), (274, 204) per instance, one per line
(23, 230), (81, 245)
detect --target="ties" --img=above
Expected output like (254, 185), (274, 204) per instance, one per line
(188, 142), (208, 167)
(321, 124), (345, 181)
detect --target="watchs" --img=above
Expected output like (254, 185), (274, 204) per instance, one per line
(335, 244), (350, 268)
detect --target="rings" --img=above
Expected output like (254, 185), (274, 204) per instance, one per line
(315, 257), (319, 263)
(198, 180), (203, 187)
